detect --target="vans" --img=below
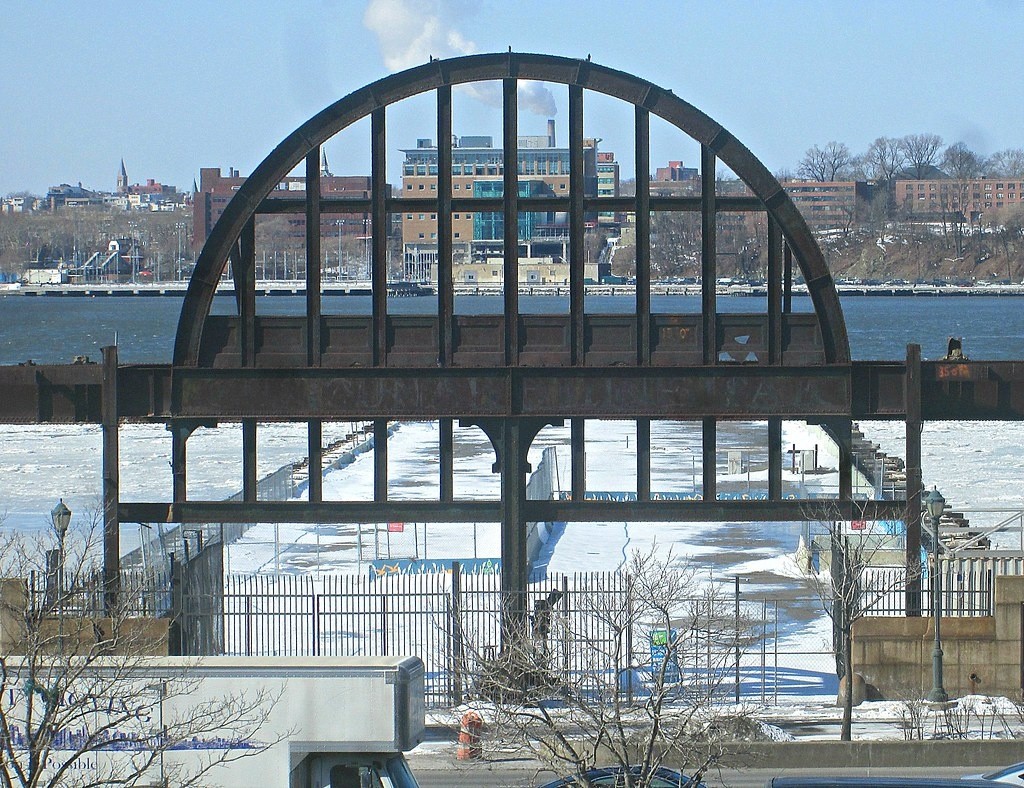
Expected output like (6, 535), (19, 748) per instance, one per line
(765, 775), (1021, 788)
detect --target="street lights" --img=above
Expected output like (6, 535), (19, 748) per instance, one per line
(335, 219), (346, 280)
(363, 219), (371, 279)
(128, 221), (139, 284)
(918, 481), (952, 703)
(175, 221), (186, 281)
(49, 498), (73, 652)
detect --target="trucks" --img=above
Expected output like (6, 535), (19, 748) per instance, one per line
(0, 653), (432, 788)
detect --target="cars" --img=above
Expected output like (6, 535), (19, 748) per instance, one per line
(601, 274), (1014, 287)
(536, 766), (707, 788)
(959, 760), (1024, 787)
